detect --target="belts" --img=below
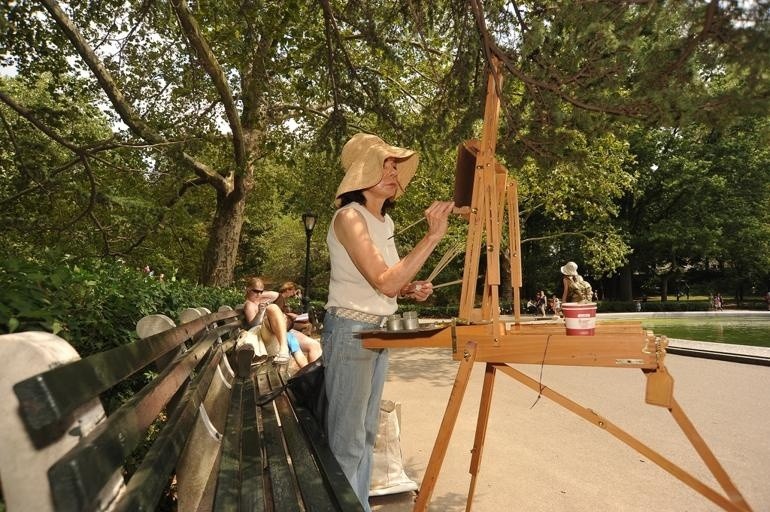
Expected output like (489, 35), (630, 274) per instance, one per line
(327, 307), (388, 327)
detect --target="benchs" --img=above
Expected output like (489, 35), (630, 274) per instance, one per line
(14, 306), (366, 510)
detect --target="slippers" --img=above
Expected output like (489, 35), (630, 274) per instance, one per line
(272, 354), (290, 365)
(238, 344), (254, 377)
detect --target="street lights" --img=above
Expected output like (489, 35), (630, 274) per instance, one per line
(300, 210), (320, 309)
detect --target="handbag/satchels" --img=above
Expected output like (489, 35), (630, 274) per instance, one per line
(255, 357), (326, 428)
(370, 399), (402, 491)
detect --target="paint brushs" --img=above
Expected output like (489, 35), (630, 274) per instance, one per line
(404, 241), (482, 298)
(387, 217), (426, 240)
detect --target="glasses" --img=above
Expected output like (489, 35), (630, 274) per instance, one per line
(252, 289), (263, 294)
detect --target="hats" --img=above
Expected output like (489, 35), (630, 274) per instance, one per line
(560, 262), (578, 276)
(334, 132), (419, 208)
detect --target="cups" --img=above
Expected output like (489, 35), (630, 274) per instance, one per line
(559, 300), (598, 336)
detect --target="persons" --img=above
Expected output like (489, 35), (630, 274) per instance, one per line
(560, 261), (594, 303)
(636, 301), (642, 311)
(642, 293), (647, 312)
(710, 292), (724, 312)
(594, 289), (599, 300)
(526, 290), (561, 318)
(318, 132), (455, 511)
(239, 276), (322, 369)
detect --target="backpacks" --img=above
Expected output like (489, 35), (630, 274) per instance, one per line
(564, 276), (593, 303)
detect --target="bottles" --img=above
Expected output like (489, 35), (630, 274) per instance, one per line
(387, 310), (420, 330)
